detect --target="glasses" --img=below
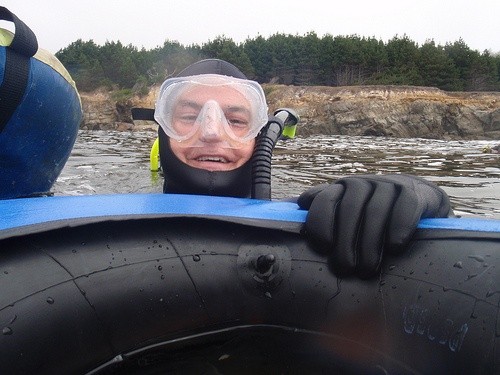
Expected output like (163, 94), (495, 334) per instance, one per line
(153, 76), (270, 142)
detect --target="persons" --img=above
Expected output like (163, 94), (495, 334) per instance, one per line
(149, 59), (449, 279)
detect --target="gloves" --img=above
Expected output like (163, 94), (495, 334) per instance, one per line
(298, 173), (450, 277)
